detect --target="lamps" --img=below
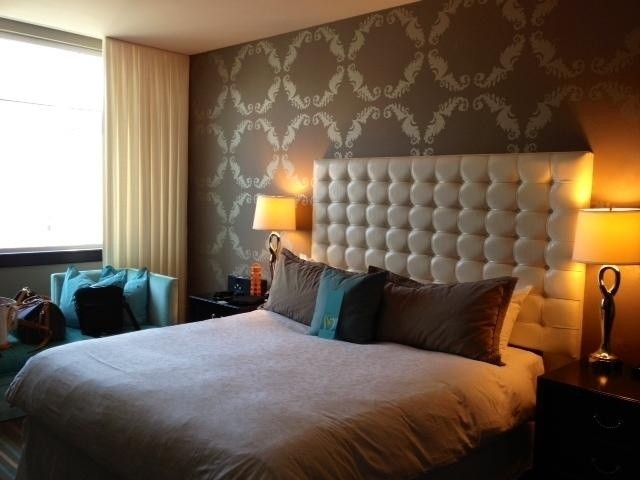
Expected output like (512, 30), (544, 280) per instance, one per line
(252, 195), (296, 297)
(573, 208), (640, 369)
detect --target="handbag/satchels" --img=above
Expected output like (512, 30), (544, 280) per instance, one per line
(9, 287), (65, 346)
(0, 296), (17, 348)
(73, 286), (124, 336)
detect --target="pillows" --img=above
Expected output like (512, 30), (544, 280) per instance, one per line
(257, 247), (535, 367)
(59, 263), (149, 329)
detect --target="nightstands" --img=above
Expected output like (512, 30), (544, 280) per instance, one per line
(533, 355), (640, 480)
(185, 291), (269, 322)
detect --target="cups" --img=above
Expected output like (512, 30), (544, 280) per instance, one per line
(250, 262), (263, 300)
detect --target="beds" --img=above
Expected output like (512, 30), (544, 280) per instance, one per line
(4, 150), (596, 479)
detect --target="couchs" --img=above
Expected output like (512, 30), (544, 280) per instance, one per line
(0, 267), (179, 422)
